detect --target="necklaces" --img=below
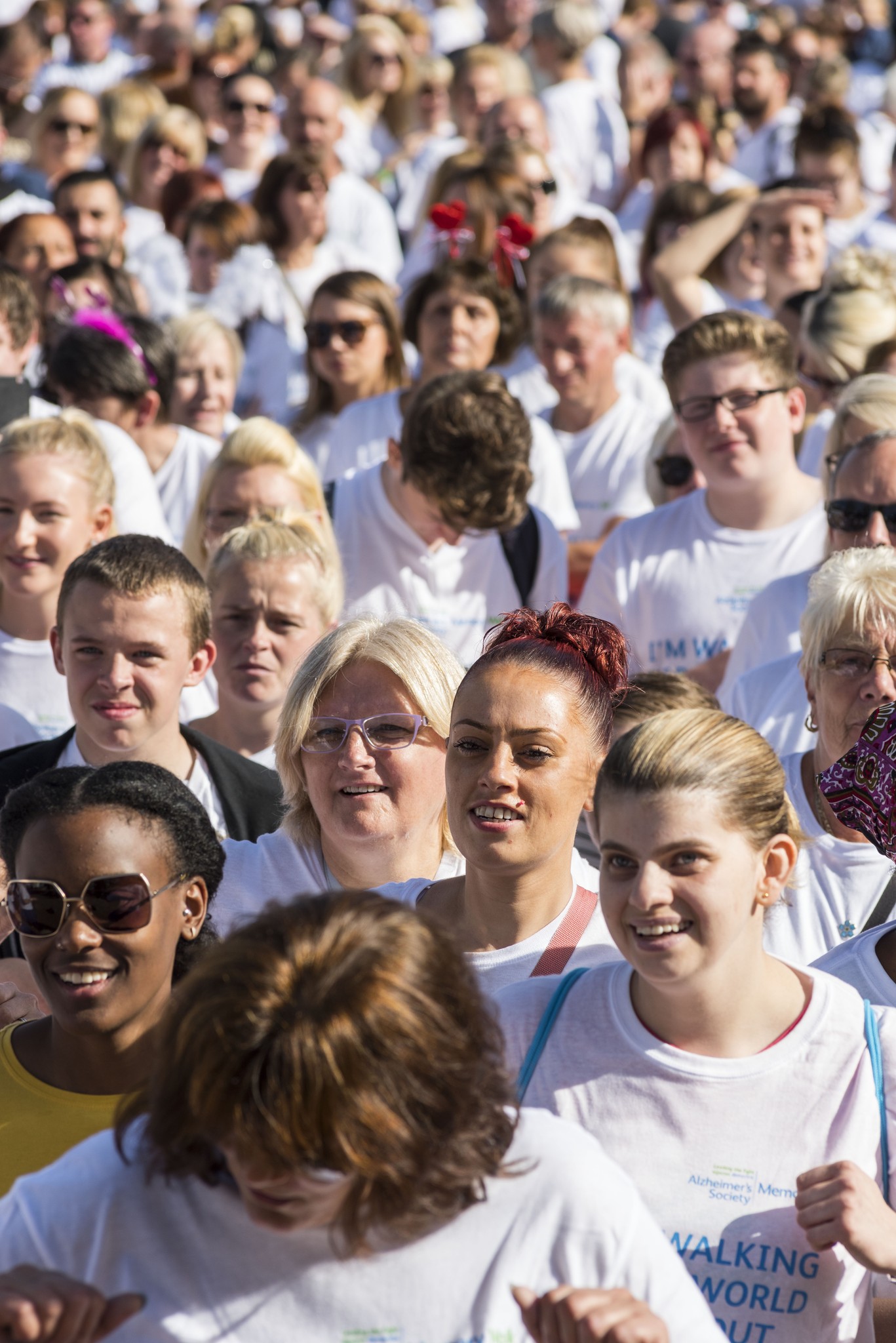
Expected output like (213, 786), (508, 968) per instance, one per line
(323, 855), (331, 892)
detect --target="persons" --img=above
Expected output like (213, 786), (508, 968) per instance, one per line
(0, 0), (896, 1343)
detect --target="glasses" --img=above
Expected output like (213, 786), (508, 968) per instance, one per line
(819, 647), (896, 675)
(52, 119), (92, 134)
(303, 319), (387, 350)
(675, 386), (789, 422)
(302, 712), (431, 752)
(1, 873), (190, 934)
(825, 498), (896, 535)
(227, 101), (272, 114)
(652, 454), (696, 488)
(825, 451), (844, 475)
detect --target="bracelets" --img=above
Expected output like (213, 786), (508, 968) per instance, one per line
(886, 1273), (896, 1284)
